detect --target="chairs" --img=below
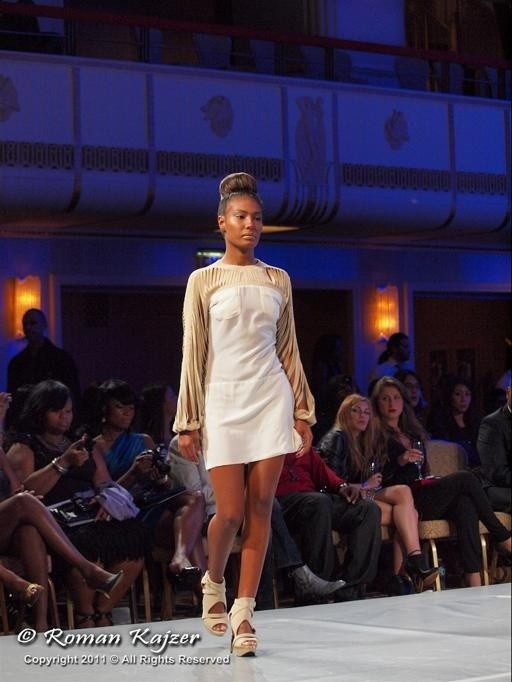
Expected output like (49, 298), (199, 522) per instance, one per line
(0, 539), (279, 632)
(418, 441), (511, 592)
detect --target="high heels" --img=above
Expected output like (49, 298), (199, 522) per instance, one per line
(92, 571), (125, 599)
(166, 566), (200, 593)
(384, 574), (413, 598)
(200, 573), (228, 637)
(406, 554), (443, 591)
(229, 599), (259, 657)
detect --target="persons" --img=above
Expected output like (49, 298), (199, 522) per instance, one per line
(72, 377), (206, 619)
(0, 391), (124, 633)
(315, 393), (447, 597)
(369, 375), (512, 588)
(370, 332), (416, 383)
(171, 172), (317, 657)
(433, 369), (487, 468)
(275, 444), (382, 606)
(476, 367), (512, 515)
(2, 379), (162, 629)
(1, 560), (47, 607)
(5, 309), (82, 389)
(393, 369), (431, 441)
(135, 383), (346, 605)
(2, 383), (38, 434)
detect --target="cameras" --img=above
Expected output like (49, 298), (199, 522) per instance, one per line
(136, 441), (171, 476)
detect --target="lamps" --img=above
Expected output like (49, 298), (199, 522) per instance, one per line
(13, 277), (41, 335)
(371, 285), (400, 338)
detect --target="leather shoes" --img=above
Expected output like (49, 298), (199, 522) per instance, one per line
(11, 581), (44, 612)
(288, 564), (347, 602)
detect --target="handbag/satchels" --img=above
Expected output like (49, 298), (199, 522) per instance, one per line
(50, 496), (112, 528)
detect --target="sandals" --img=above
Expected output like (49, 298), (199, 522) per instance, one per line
(95, 615), (113, 627)
(75, 613), (95, 628)
